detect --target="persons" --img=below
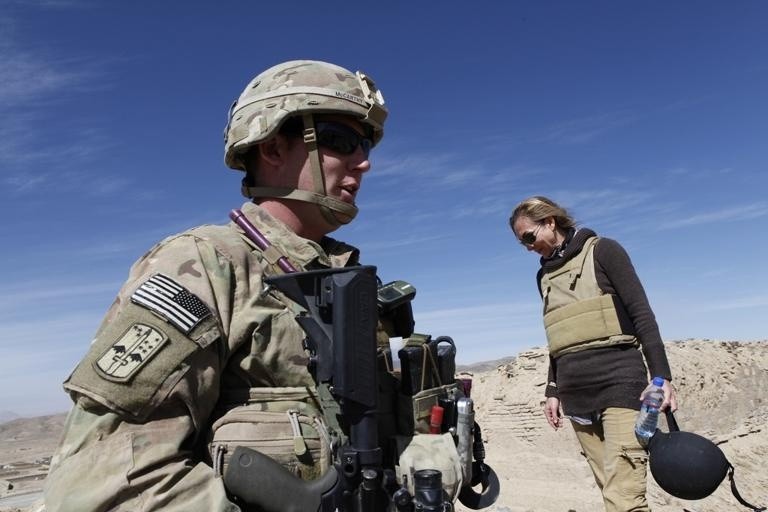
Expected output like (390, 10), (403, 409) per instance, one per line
(509, 196), (677, 512)
(41, 58), (486, 510)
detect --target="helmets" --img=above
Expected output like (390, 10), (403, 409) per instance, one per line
(223, 58), (390, 174)
(647, 429), (730, 501)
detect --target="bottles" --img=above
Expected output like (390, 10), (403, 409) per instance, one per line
(458, 378), (472, 399)
(634, 376), (664, 437)
(431, 406), (444, 434)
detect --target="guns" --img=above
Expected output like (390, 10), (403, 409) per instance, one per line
(222, 263), (444, 511)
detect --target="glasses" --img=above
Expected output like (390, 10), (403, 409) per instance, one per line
(520, 223), (544, 245)
(297, 122), (372, 161)
